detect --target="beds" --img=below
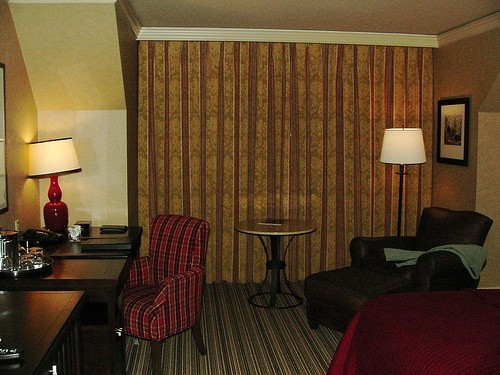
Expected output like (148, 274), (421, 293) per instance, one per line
(327, 289), (500, 374)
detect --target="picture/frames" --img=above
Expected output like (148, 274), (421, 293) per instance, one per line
(437, 97), (471, 165)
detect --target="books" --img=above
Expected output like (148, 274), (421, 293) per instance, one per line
(258, 219), (283, 227)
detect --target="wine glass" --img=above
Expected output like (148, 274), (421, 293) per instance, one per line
(0, 238), (13, 271)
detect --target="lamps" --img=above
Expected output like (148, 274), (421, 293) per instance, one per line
(27, 137), (82, 234)
(379, 128), (427, 238)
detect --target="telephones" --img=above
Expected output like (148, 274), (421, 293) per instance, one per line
(22, 227), (66, 248)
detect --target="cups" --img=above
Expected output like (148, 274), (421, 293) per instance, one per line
(67, 224), (82, 242)
(20, 247), (44, 271)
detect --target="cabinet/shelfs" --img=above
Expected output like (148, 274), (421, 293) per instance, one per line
(0, 290), (89, 375)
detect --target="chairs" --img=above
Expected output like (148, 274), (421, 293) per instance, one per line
(122, 214), (215, 375)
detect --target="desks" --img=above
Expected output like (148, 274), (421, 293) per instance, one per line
(236, 219), (316, 309)
(0, 224), (142, 375)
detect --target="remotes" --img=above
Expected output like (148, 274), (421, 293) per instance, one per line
(0, 348), (20, 360)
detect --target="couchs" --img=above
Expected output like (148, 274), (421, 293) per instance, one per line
(305, 207), (492, 333)
